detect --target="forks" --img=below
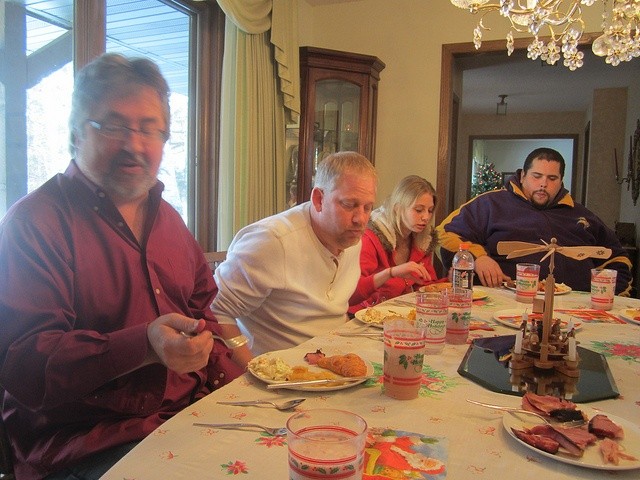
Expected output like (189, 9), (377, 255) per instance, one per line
(192, 422), (288, 436)
(466, 398), (585, 429)
(181, 331), (249, 349)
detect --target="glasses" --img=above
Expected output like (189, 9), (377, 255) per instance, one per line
(89, 120), (168, 145)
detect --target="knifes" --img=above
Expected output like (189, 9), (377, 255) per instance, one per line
(266, 376), (362, 388)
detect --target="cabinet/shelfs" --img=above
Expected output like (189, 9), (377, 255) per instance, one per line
(299, 46), (385, 204)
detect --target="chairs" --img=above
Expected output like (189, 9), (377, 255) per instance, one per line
(203, 250), (229, 278)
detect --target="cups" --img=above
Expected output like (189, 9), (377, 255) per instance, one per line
(382, 320), (426, 400)
(620, 307), (640, 325)
(516, 263), (540, 303)
(286, 410), (367, 480)
(416, 292), (448, 348)
(591, 268), (617, 311)
(445, 287), (473, 344)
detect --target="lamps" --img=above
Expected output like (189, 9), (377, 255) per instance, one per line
(614, 119), (639, 207)
(450, 0), (640, 72)
(496, 95), (510, 116)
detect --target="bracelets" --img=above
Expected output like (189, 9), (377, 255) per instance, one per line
(388, 266), (394, 279)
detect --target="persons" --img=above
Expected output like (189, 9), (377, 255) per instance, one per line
(347, 174), (448, 319)
(209, 151), (377, 372)
(0, 53), (246, 480)
(434, 148), (634, 297)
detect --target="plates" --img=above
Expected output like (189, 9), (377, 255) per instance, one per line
(503, 278), (572, 295)
(418, 283), (487, 301)
(504, 402), (640, 471)
(246, 350), (375, 391)
(493, 309), (583, 332)
(354, 305), (420, 330)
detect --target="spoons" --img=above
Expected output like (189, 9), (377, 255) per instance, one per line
(217, 397), (305, 411)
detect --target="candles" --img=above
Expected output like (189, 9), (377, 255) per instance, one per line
(615, 147), (619, 176)
(629, 136), (633, 170)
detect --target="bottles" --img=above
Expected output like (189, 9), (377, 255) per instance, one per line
(452, 244), (474, 293)
